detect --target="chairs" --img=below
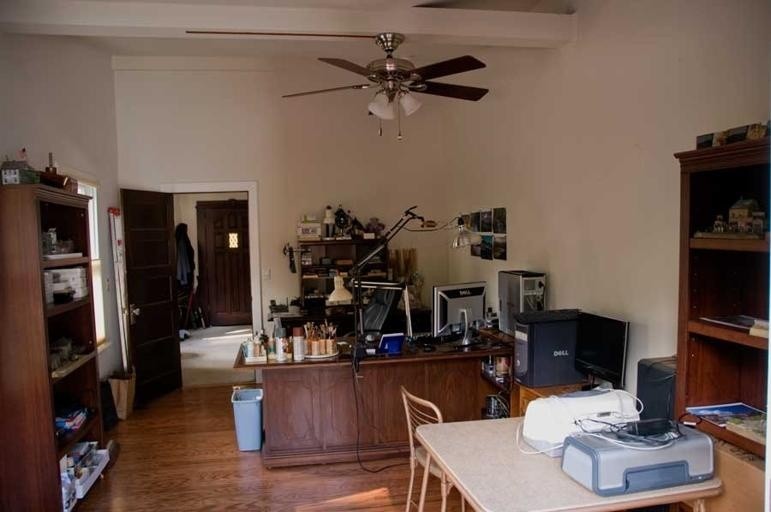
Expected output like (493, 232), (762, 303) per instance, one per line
(399, 385), (465, 512)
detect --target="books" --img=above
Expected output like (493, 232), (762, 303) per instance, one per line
(55, 407), (89, 431)
(750, 317), (769, 339)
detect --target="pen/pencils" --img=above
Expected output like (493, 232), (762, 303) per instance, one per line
(304, 318), (339, 340)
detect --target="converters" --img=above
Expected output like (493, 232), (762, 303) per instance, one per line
(626, 419), (672, 436)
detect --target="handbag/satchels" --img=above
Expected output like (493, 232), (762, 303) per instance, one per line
(107, 366), (136, 420)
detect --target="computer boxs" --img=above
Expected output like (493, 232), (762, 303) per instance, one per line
(512, 308), (578, 388)
(498, 270), (547, 338)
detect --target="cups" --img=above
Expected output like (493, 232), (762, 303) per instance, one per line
(487, 395), (501, 416)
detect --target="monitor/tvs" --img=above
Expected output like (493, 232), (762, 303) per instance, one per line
(431, 280), (488, 345)
(574, 311), (630, 390)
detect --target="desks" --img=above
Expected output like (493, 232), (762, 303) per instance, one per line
(510, 382), (587, 417)
(261, 333), (512, 469)
(414, 417), (724, 512)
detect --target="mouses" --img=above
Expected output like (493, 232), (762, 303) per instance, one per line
(423, 345), (435, 351)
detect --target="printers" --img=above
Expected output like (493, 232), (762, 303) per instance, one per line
(560, 415), (715, 496)
(521, 389), (641, 459)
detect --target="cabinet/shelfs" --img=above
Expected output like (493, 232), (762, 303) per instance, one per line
(0, 183), (104, 512)
(296, 238), (390, 323)
(674, 138), (770, 465)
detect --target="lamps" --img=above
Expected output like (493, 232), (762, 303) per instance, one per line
(325, 272), (415, 339)
(368, 84), (425, 142)
(348, 206), (482, 358)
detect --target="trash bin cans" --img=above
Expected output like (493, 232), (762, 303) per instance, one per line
(231, 389), (263, 452)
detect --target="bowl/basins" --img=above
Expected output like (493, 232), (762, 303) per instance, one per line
(30, 170), (69, 189)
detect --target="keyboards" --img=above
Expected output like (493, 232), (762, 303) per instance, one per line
(416, 326), (480, 348)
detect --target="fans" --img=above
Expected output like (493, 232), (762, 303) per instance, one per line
(281, 33), (491, 102)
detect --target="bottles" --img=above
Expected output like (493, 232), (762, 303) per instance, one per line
(275, 327), (304, 361)
